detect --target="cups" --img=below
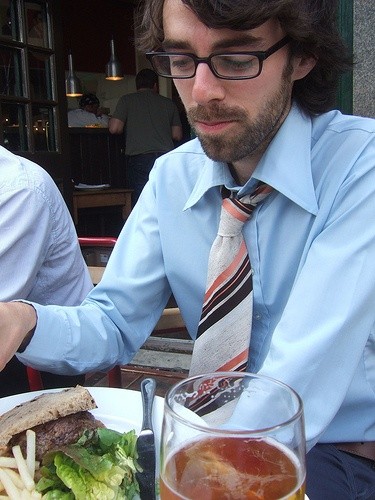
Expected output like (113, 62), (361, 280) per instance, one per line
(159, 372), (308, 499)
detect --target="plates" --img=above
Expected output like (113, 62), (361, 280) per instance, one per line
(0, 387), (210, 500)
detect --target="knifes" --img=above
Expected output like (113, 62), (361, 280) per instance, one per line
(133, 377), (157, 500)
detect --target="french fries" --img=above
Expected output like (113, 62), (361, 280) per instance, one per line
(0, 428), (45, 500)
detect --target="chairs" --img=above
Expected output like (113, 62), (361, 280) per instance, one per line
(27, 238), (121, 391)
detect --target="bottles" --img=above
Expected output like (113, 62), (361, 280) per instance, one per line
(35, 120), (47, 151)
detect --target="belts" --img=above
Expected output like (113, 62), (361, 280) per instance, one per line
(332, 442), (375, 461)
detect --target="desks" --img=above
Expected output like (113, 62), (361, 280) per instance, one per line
(72, 188), (134, 227)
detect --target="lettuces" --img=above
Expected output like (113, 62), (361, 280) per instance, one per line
(35, 426), (142, 500)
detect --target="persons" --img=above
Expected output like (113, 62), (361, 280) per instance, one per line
(0, 0), (374, 500)
(0, 143), (95, 402)
(68, 79), (110, 128)
(109, 68), (183, 214)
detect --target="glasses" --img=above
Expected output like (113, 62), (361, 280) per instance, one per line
(145, 35), (291, 81)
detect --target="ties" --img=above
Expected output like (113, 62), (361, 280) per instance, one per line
(184, 180), (275, 424)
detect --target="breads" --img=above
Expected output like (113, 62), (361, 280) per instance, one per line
(0, 385), (98, 450)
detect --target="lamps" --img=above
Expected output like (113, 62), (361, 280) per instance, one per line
(66, 53), (82, 97)
(104, 36), (124, 81)
